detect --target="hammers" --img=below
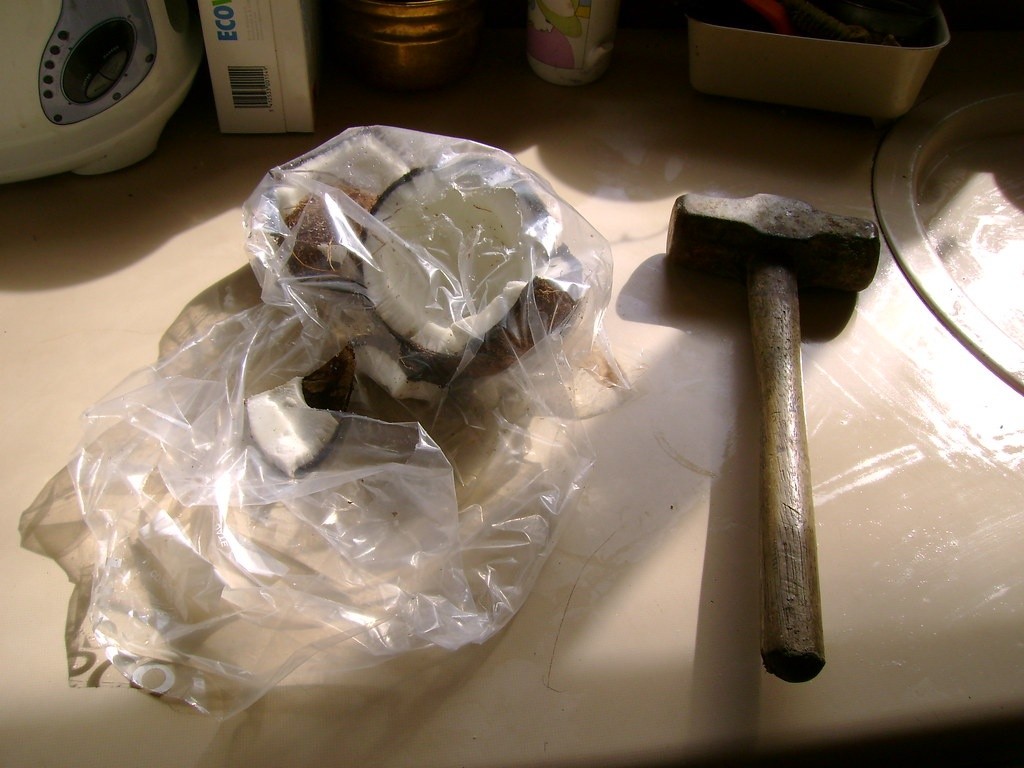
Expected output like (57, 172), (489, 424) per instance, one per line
(660, 186), (884, 683)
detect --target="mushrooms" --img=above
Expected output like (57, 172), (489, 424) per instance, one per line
(240, 131), (584, 472)
